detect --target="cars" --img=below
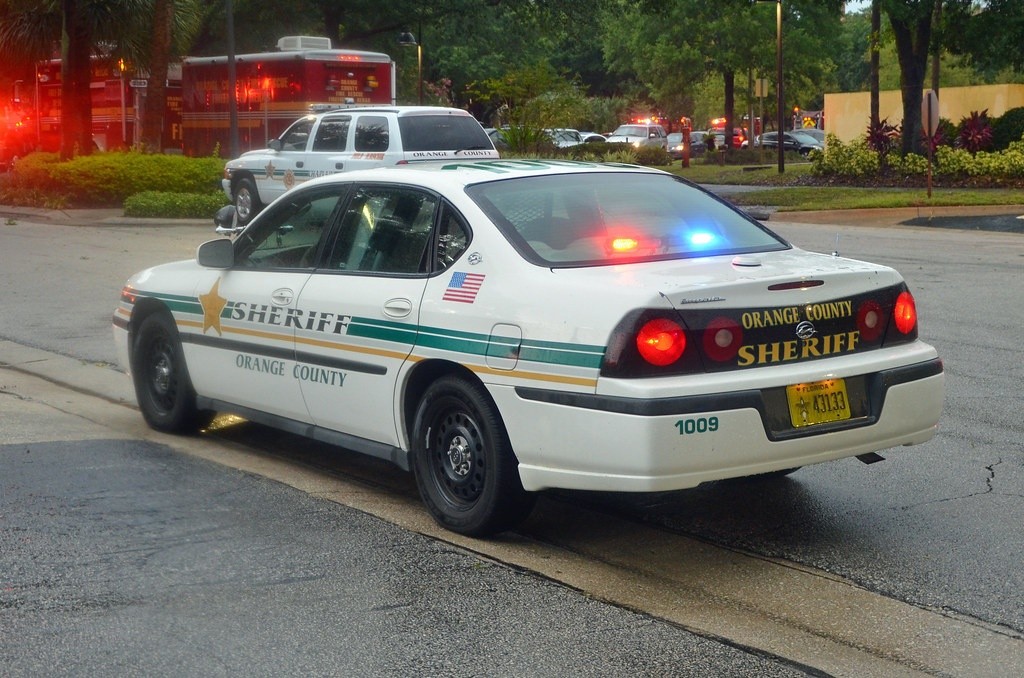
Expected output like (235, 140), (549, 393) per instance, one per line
(665, 133), (685, 160)
(707, 127), (726, 150)
(605, 125), (668, 150)
(221, 98), (500, 226)
(485, 127), (583, 154)
(792, 129), (824, 145)
(111, 158), (944, 537)
(690, 132), (714, 158)
(713, 128), (749, 148)
(577, 132), (607, 143)
(741, 132), (824, 160)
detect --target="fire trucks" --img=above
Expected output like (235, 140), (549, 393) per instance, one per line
(11, 35), (395, 159)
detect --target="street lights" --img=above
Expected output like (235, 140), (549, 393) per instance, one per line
(397, 32), (422, 105)
